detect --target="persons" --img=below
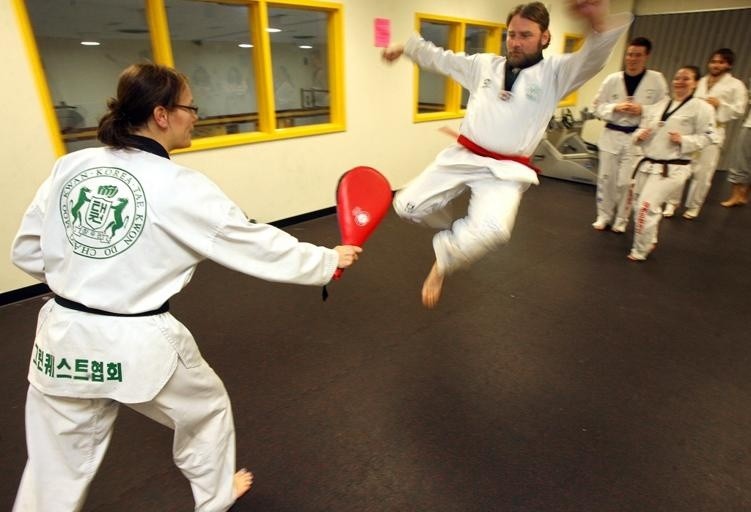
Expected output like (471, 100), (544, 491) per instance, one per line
(621, 61), (720, 260)
(380, 1), (638, 312)
(720, 110), (751, 209)
(587, 36), (671, 235)
(661, 47), (749, 222)
(10, 61), (365, 512)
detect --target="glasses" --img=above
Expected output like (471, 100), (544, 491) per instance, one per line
(167, 103), (198, 116)
